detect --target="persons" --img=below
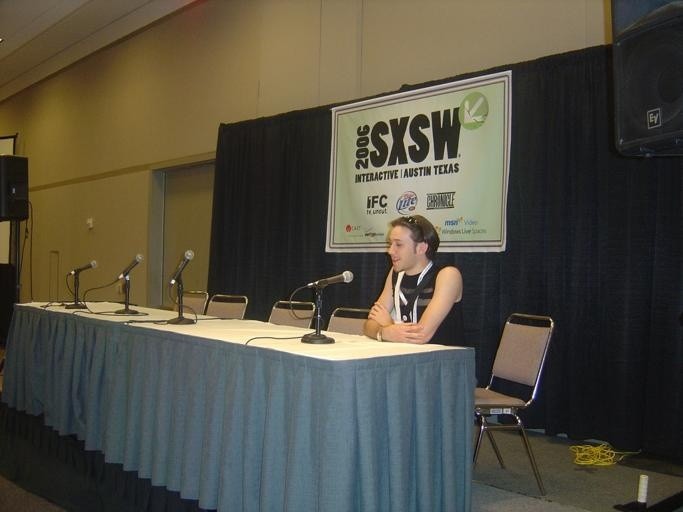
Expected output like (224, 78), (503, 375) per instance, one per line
(363, 214), (467, 348)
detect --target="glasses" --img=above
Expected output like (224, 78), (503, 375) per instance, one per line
(406, 216), (418, 225)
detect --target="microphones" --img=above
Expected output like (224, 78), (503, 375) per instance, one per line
(118, 253), (144, 280)
(170, 249), (195, 287)
(70, 260), (99, 275)
(307, 271), (354, 289)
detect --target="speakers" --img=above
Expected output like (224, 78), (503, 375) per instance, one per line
(611, 0), (683, 156)
(0, 154), (29, 222)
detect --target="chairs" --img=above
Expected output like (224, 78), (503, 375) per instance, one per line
(206, 294), (249, 319)
(327, 307), (370, 336)
(174, 290), (209, 314)
(473, 313), (555, 496)
(269, 301), (315, 328)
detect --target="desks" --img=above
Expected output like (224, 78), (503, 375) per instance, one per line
(0, 302), (476, 512)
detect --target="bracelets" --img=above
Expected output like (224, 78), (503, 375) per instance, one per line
(376, 326), (383, 342)
